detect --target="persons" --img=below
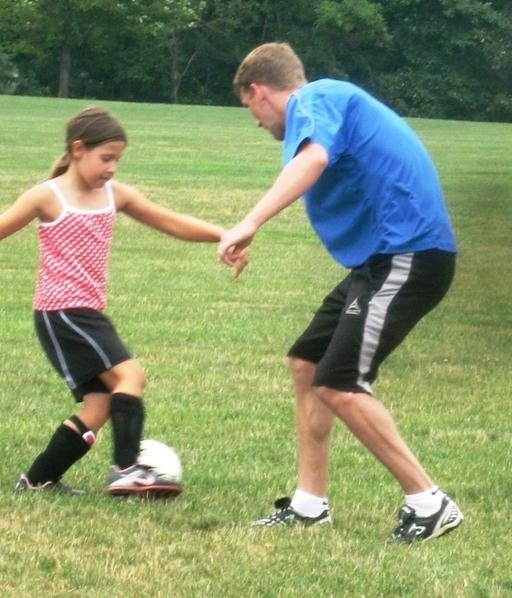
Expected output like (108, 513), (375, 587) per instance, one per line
(215, 42), (464, 544)
(0, 106), (232, 496)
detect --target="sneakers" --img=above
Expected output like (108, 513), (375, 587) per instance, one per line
(388, 496), (464, 548)
(13, 474), (86, 497)
(103, 464), (182, 496)
(251, 498), (334, 530)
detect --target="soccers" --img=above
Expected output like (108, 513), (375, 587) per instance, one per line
(136, 438), (184, 481)
